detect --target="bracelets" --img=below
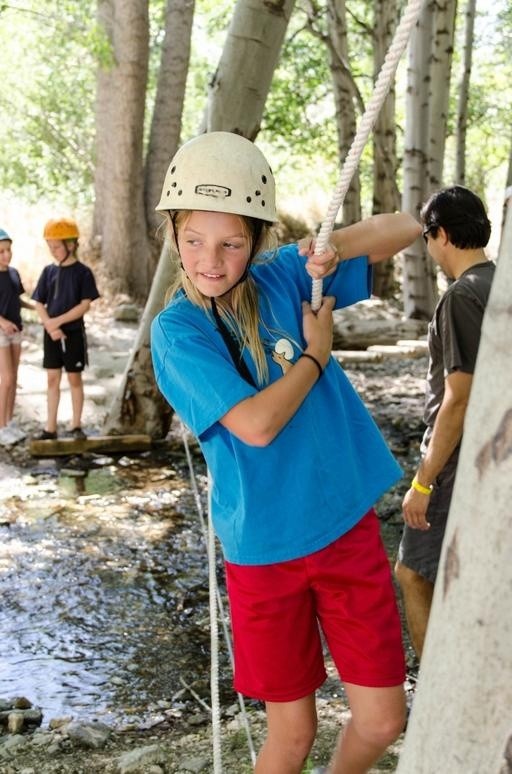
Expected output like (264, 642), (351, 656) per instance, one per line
(411, 476), (434, 496)
(297, 354), (324, 385)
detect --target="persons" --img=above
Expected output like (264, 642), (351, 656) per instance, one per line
(152, 130), (423, 774)
(1, 229), (36, 445)
(29, 218), (102, 440)
(393, 184), (496, 660)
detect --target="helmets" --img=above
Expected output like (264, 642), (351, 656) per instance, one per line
(42, 217), (80, 241)
(0, 229), (13, 243)
(155, 131), (278, 227)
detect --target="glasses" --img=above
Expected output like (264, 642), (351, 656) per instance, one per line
(423, 230), (430, 242)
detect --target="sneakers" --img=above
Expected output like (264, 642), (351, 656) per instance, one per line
(0, 419), (26, 446)
(33, 430), (57, 439)
(68, 426), (87, 441)
